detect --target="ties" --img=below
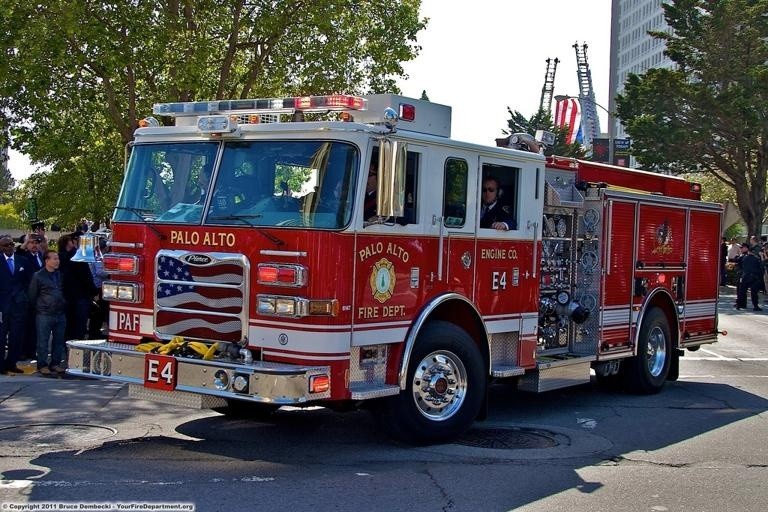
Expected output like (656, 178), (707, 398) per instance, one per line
(7, 257), (14, 274)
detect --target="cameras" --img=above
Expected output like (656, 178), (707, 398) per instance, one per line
(32, 235), (42, 241)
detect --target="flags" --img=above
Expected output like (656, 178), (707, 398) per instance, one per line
(554, 97), (583, 146)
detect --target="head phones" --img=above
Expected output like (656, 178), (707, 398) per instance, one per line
(497, 179), (505, 199)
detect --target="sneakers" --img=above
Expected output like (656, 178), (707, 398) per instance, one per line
(39, 365), (66, 374)
(0, 354), (35, 374)
(734, 303), (762, 311)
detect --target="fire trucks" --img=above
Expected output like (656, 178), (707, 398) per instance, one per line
(73, 41), (725, 447)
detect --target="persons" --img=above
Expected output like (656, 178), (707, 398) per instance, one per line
(720, 234), (768, 310)
(191, 163), (246, 215)
(0, 222), (109, 376)
(480, 177), (516, 230)
(364, 173), (378, 220)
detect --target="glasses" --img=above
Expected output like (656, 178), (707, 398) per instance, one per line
(1, 243), (15, 247)
(482, 188), (497, 192)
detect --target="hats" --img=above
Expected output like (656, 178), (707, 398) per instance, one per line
(740, 243), (755, 252)
(32, 223), (44, 230)
(368, 164), (377, 176)
